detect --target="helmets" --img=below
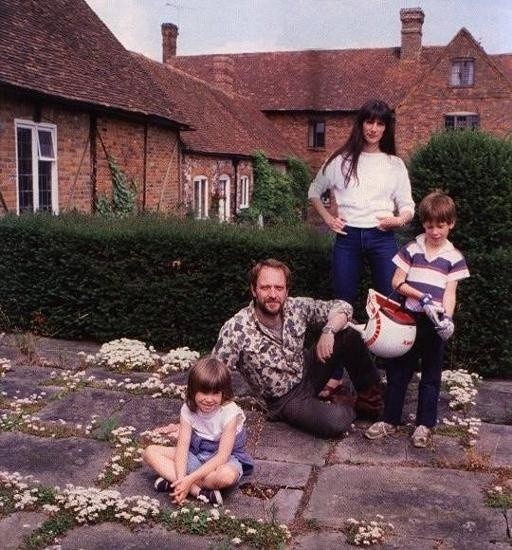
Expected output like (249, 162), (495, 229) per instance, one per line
(353, 289), (417, 358)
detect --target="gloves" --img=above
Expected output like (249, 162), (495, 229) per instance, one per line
(419, 293), (454, 340)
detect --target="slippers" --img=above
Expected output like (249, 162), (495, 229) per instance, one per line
(320, 384), (342, 398)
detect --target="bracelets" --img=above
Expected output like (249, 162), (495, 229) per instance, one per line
(397, 215), (405, 227)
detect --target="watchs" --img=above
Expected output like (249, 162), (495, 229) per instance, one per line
(321, 325), (337, 334)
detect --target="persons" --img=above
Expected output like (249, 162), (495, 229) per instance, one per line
(308, 100), (417, 402)
(364, 193), (471, 448)
(212, 258), (383, 436)
(140, 357), (255, 509)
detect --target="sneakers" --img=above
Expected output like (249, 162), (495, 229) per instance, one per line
(197, 490), (223, 505)
(410, 425), (432, 447)
(365, 422), (399, 439)
(154, 477), (173, 491)
